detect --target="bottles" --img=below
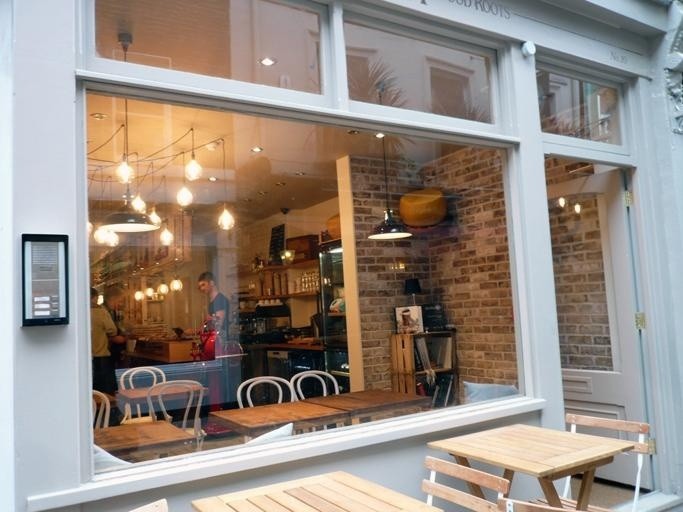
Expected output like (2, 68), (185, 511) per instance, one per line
(254, 276), (262, 297)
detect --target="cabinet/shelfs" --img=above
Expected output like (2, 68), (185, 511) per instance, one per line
(224, 256), (320, 301)
(390, 328), (458, 403)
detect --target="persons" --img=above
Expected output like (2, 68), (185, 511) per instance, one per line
(101, 287), (129, 368)
(90, 287), (118, 393)
(397, 307), (421, 334)
(183, 272), (230, 345)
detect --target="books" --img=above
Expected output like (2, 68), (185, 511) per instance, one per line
(391, 300), (455, 412)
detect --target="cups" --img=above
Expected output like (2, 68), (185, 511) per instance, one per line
(264, 270), (299, 296)
(258, 299), (282, 306)
(300, 270), (320, 292)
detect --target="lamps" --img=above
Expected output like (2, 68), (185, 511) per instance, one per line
(368, 134), (412, 239)
(404, 278), (422, 305)
(88, 32), (235, 303)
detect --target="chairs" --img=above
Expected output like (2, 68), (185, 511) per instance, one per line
(421, 456), (513, 512)
(485, 495), (574, 512)
(562, 410), (655, 510)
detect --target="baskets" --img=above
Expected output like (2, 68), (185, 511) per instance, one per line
(326, 215), (340, 240)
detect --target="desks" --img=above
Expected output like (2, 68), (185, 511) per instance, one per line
(425, 422), (636, 508)
(188, 472), (450, 510)
